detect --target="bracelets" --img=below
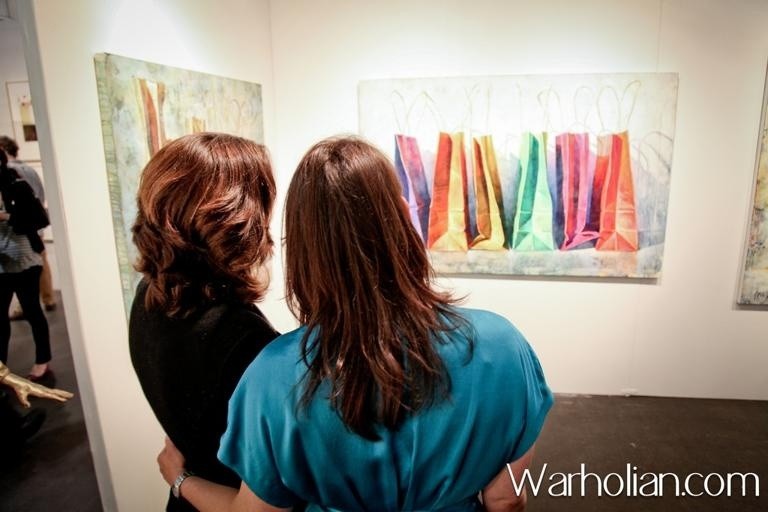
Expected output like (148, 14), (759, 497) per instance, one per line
(171, 470), (201, 500)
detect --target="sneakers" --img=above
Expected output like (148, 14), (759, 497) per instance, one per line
(10, 310), (26, 320)
(45, 304), (57, 312)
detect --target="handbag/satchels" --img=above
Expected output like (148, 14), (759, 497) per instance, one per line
(395, 131), (639, 252)
(6, 199), (50, 235)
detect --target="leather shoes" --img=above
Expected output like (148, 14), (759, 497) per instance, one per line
(26, 364), (49, 381)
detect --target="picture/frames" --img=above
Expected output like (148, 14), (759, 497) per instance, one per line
(6, 80), (42, 162)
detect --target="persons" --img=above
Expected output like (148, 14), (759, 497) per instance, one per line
(157, 133), (554, 511)
(1, 148), (54, 383)
(1, 135), (57, 323)
(0, 360), (75, 409)
(123, 132), (310, 509)
